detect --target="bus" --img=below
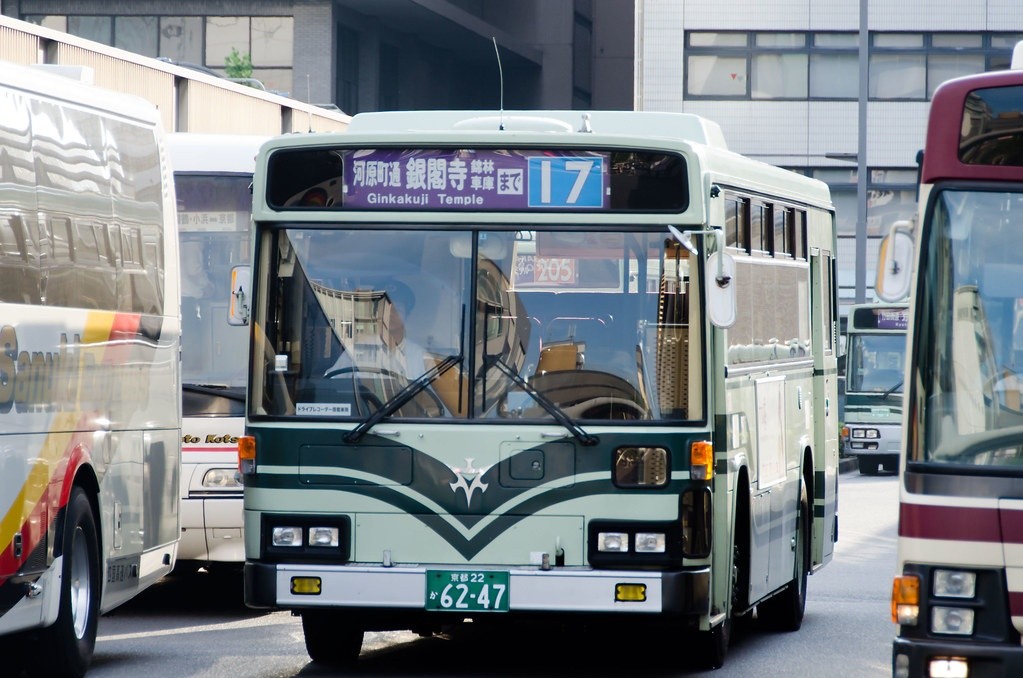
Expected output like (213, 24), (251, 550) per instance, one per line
(836, 301), (910, 475)
(224, 109), (841, 678)
(871, 67), (1023, 678)
(136, 130), (285, 607)
(0, 56), (185, 678)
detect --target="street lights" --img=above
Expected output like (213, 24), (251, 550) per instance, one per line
(955, 284), (1001, 431)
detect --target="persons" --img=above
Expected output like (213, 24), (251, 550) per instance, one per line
(322, 280), (440, 392)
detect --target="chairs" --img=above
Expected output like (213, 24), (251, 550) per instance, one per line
(543, 311), (615, 351)
(484, 314), (541, 384)
